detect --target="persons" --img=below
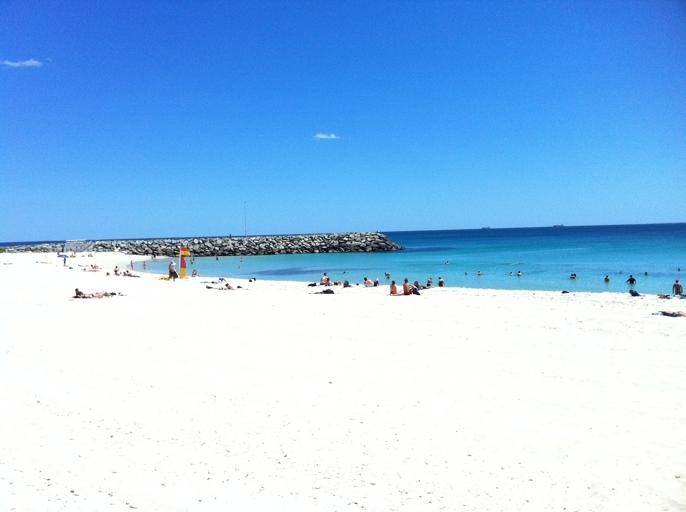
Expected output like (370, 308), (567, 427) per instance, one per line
(626, 275), (636, 284)
(604, 274), (610, 282)
(570, 273), (576, 280)
(72, 260), (198, 298)
(672, 279), (683, 295)
(319, 271), (444, 295)
(509, 270), (522, 276)
